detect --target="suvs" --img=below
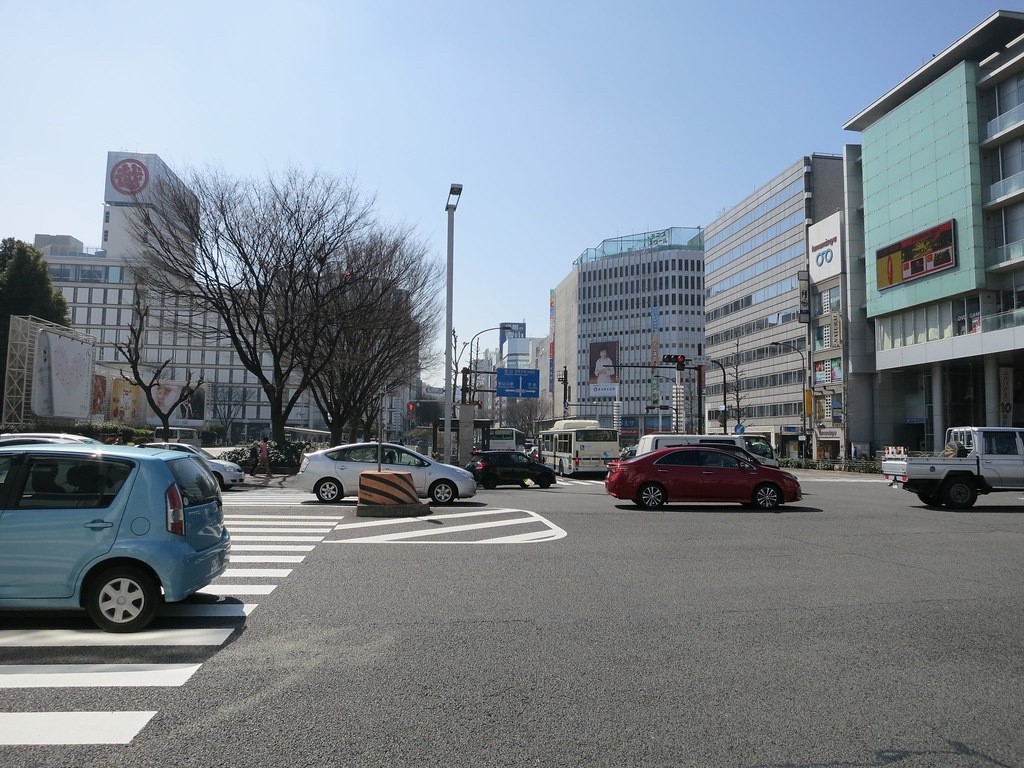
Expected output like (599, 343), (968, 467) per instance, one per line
(465, 450), (556, 490)
(133, 444), (245, 494)
(1, 431), (107, 446)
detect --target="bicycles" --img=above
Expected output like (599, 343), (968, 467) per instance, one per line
(216, 441), (330, 467)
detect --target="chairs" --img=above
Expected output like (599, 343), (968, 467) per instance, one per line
(63, 466), (105, 509)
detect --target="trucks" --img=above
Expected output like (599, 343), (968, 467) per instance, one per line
(882, 426), (1024, 511)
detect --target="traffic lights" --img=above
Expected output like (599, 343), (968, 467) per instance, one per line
(663, 355), (685, 363)
(407, 403), (415, 412)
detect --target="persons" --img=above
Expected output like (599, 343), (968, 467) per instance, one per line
(396, 438), (404, 463)
(299, 443), (314, 464)
(253, 436), (275, 479)
(146, 381), (181, 420)
(529, 449), (538, 460)
(592, 348), (617, 385)
(383, 449), (399, 463)
(249, 440), (259, 477)
(103, 436), (125, 446)
(746, 437), (756, 452)
(176, 385), (195, 419)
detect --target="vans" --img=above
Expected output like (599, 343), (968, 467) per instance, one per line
(636, 434), (780, 471)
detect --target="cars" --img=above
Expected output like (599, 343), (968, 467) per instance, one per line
(296, 439), (478, 509)
(527, 446), (538, 461)
(0, 441), (231, 634)
(620, 450), (637, 461)
(605, 446), (803, 510)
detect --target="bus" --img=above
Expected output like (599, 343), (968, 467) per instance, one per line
(438, 417), (493, 465)
(545, 440), (568, 452)
(526, 438), (537, 445)
(490, 428), (526, 452)
(538, 420), (620, 477)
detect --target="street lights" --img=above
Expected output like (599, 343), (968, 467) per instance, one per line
(770, 341), (805, 460)
(443, 182), (463, 465)
(654, 375), (678, 434)
(469, 326), (512, 404)
